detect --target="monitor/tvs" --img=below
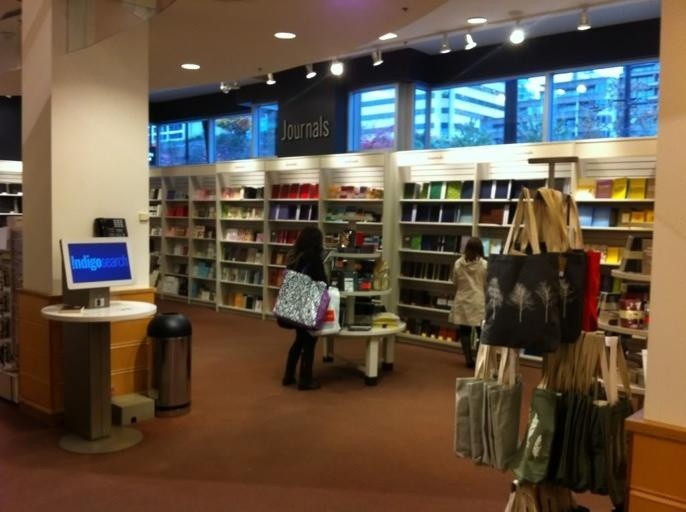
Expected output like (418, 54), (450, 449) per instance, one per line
(60, 237), (135, 291)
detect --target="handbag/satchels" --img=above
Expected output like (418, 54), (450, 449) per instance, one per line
(521, 330), (641, 508)
(306, 284), (342, 337)
(452, 341), (522, 472)
(271, 269), (331, 330)
(479, 186), (603, 349)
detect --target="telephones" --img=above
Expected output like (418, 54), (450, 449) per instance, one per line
(95, 218), (128, 237)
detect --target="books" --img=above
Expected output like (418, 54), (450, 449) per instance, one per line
(0, 183), (22, 228)
(269, 183), (318, 288)
(397, 178), (564, 347)
(150, 186), (263, 312)
(576, 176), (655, 390)
(323, 185), (382, 279)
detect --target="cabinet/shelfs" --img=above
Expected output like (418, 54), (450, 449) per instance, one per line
(0, 176), (24, 218)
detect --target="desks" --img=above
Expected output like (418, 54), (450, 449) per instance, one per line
(38, 300), (158, 456)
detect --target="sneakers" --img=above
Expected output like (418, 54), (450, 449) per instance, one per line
(282, 378), (298, 386)
(297, 382), (321, 390)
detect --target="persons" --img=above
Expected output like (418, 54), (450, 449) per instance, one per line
(448, 237), (488, 368)
(276, 227), (330, 389)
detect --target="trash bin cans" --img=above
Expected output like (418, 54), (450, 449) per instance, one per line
(146, 311), (192, 416)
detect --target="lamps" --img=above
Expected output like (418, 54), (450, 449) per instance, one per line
(218, 8), (592, 101)
